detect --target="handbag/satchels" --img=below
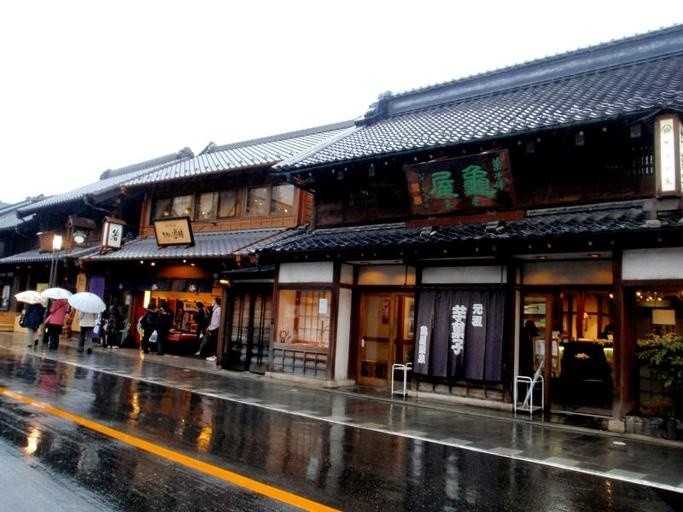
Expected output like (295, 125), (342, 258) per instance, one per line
(19, 314), (28, 327)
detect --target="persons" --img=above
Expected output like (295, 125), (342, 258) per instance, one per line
(19, 303), (42, 347)
(105, 298), (123, 350)
(75, 310), (99, 355)
(192, 296), (222, 361)
(43, 298), (69, 350)
(15, 353), (218, 436)
(139, 300), (158, 355)
(191, 301), (205, 336)
(155, 302), (174, 356)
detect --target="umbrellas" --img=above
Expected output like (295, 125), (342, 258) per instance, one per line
(12, 288), (42, 306)
(66, 291), (107, 316)
(38, 285), (72, 301)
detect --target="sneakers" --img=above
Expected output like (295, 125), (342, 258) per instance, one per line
(28, 339), (58, 351)
(195, 351), (216, 362)
(77, 348), (92, 355)
(107, 346), (165, 356)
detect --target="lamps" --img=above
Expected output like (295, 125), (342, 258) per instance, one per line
(651, 307), (676, 337)
